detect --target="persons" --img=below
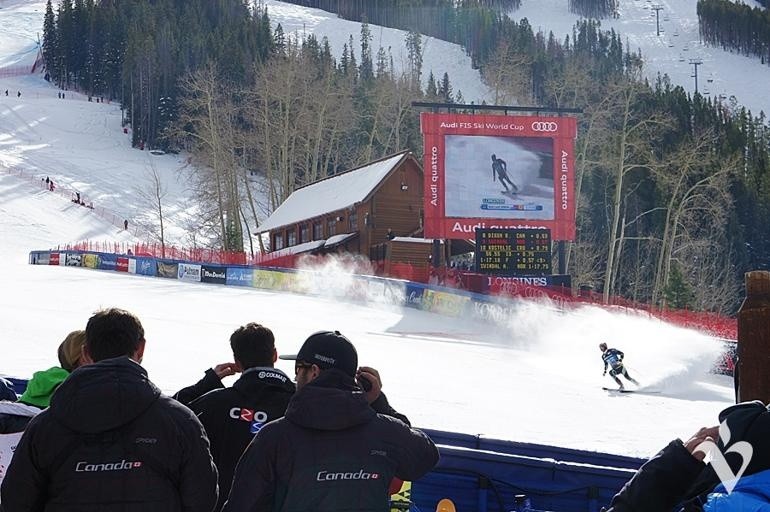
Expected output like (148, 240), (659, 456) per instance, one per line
(0, 308), (219, 511)
(18, 329), (96, 416)
(384, 226), (396, 243)
(602, 399), (770, 512)
(490, 154), (519, 196)
(600, 342), (641, 392)
(219, 328), (440, 512)
(171, 321), (301, 512)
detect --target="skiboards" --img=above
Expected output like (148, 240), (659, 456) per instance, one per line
(603, 387), (660, 394)
(501, 190), (534, 196)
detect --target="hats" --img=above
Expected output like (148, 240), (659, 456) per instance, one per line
(277, 330), (359, 378)
